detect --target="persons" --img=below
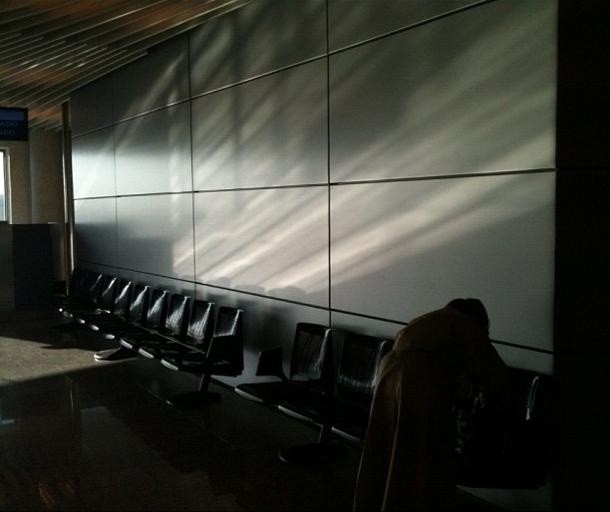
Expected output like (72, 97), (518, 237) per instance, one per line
(352, 297), (510, 509)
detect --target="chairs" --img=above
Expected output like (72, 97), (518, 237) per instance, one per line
(36, 267), (244, 412)
